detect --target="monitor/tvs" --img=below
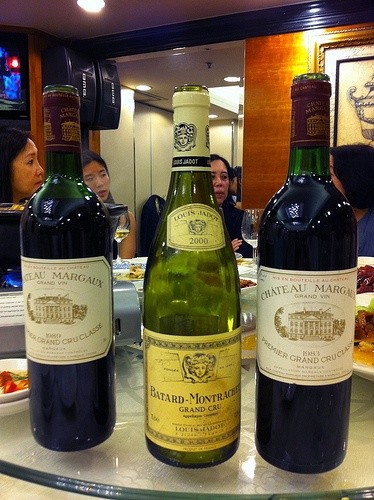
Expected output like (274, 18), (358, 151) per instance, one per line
(0, 29), (29, 114)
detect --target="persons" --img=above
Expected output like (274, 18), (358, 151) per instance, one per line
(228, 167), (238, 199)
(328, 142), (374, 258)
(81, 151), (136, 260)
(193, 153), (255, 258)
(0, 127), (46, 287)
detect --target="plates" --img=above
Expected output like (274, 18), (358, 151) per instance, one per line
(352, 292), (374, 383)
(0, 358), (29, 402)
(111, 262), (146, 281)
(233, 251), (257, 292)
(357, 256), (374, 269)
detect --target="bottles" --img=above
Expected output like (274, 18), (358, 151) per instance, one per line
(143, 84), (243, 470)
(18, 84), (117, 453)
(254, 73), (358, 475)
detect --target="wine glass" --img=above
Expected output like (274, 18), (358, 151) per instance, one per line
(241, 209), (265, 272)
(114, 212), (130, 263)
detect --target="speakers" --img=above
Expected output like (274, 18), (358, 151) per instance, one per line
(91, 57), (121, 130)
(54, 43), (98, 132)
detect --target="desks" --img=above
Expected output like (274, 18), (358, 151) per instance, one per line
(0, 256), (374, 499)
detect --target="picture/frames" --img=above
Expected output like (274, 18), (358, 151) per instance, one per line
(307, 24), (374, 144)
